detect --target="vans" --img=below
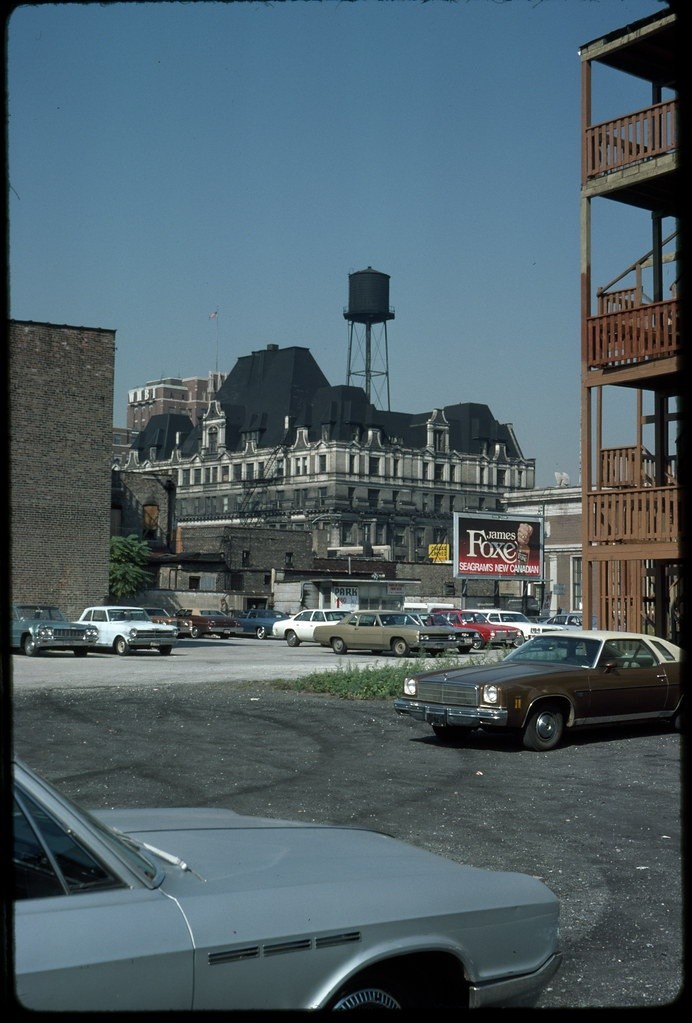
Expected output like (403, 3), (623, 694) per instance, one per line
(398, 603), (454, 614)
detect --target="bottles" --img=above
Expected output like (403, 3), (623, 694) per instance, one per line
(516, 544), (530, 576)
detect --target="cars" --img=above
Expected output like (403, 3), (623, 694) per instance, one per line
(11, 603), (99, 656)
(543, 614), (598, 631)
(272, 608), (352, 646)
(313, 610), (460, 658)
(143, 607), (193, 639)
(231, 609), (290, 639)
(527, 615), (553, 624)
(427, 610), (524, 650)
(68, 606), (178, 655)
(11, 750), (567, 1017)
(172, 607), (236, 639)
(404, 612), (481, 656)
(462, 608), (562, 644)
(402, 627), (682, 748)
